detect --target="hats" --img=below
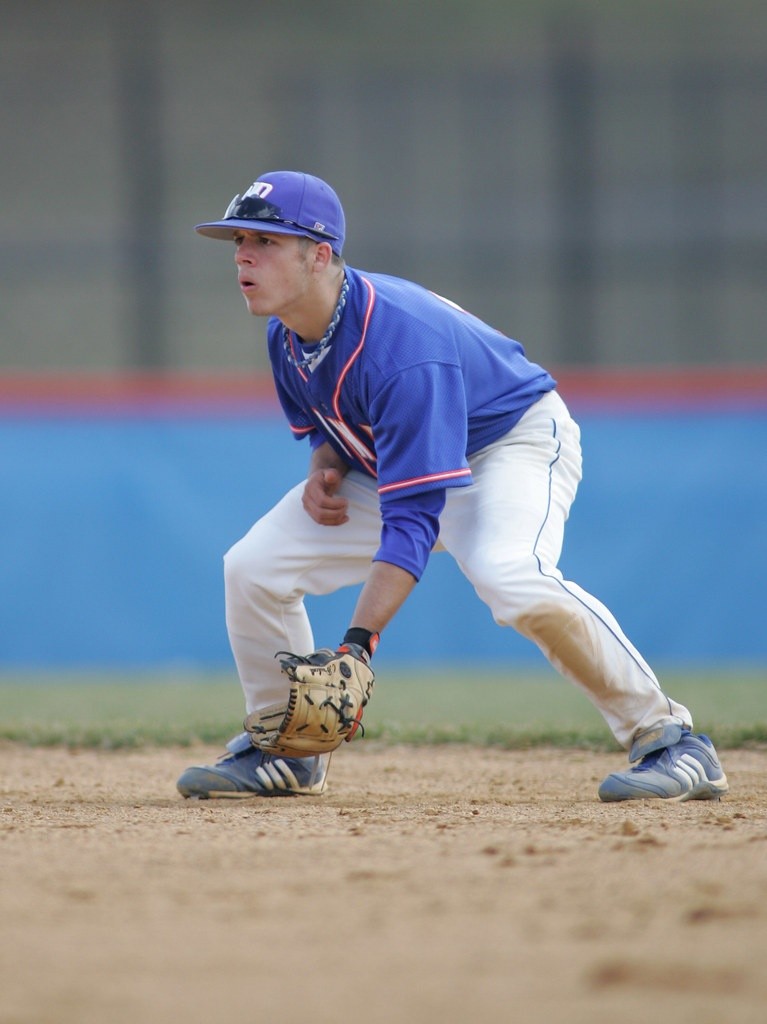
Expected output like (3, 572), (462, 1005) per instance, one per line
(193, 170), (346, 257)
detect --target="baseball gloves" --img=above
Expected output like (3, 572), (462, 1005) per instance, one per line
(241, 626), (381, 760)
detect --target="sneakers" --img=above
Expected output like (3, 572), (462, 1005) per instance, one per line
(599, 725), (729, 803)
(175, 731), (328, 799)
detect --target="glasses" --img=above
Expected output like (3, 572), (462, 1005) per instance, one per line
(222, 193), (338, 242)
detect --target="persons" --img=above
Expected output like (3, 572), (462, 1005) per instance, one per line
(176, 166), (738, 803)
